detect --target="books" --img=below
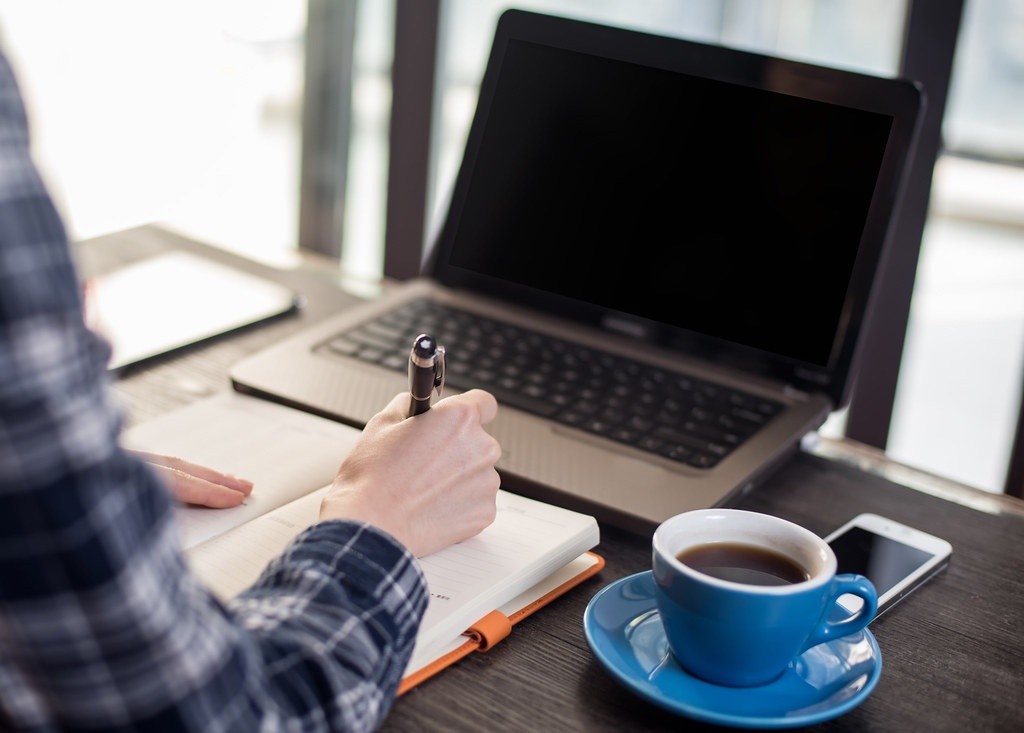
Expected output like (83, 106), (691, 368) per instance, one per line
(121, 388), (606, 696)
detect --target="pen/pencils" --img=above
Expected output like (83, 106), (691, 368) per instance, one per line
(406, 332), (447, 421)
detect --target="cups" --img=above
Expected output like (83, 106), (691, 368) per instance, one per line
(652, 509), (878, 688)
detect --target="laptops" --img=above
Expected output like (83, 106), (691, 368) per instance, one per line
(227, 10), (927, 540)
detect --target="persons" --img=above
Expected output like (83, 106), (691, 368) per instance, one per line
(0, 39), (501, 733)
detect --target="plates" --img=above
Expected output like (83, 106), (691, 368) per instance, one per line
(583, 569), (883, 729)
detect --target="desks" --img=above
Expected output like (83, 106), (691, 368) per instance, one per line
(72, 223), (1024, 733)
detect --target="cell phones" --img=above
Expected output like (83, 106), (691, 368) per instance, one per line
(821, 513), (956, 623)
